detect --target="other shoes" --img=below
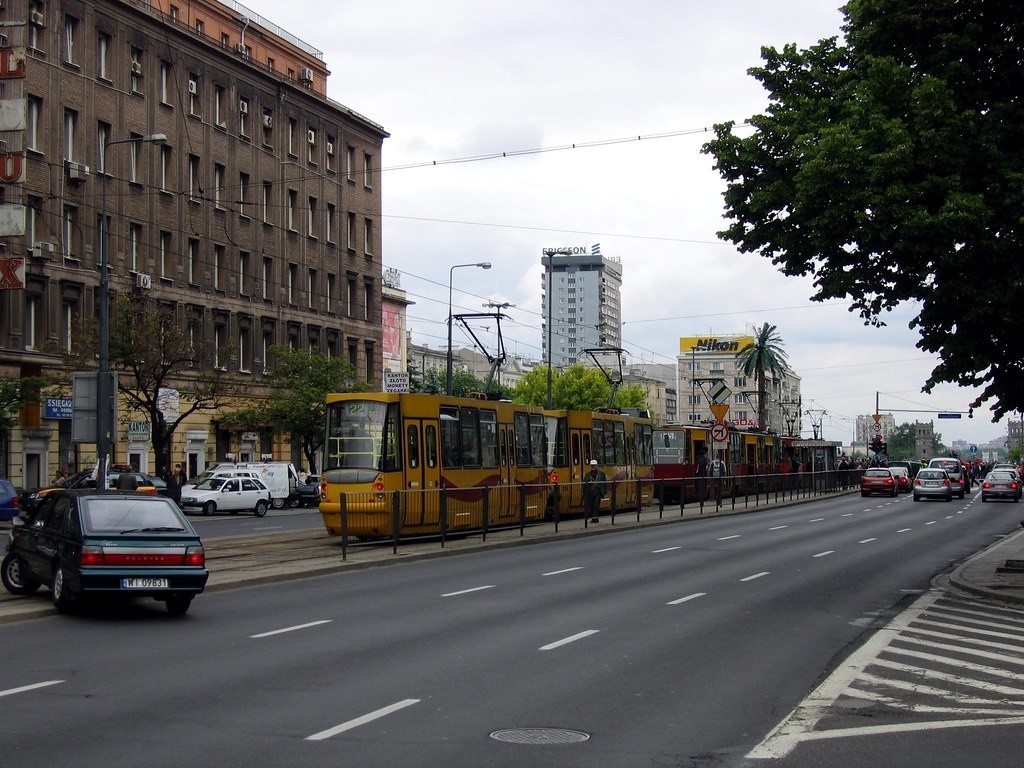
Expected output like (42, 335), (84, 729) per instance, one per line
(590, 518), (599, 523)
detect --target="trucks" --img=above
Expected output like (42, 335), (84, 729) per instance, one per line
(206, 462), (289, 510)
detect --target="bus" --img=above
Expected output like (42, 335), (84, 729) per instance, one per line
(318, 313), (827, 544)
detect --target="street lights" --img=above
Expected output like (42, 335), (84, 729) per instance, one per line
(482, 302), (517, 395)
(446, 261), (493, 397)
(96, 134), (169, 487)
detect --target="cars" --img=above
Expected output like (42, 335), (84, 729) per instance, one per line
(961, 465), (970, 494)
(991, 462), (1023, 500)
(0, 480), (20, 520)
(180, 469), (263, 492)
(889, 467), (913, 493)
(1, 488), (210, 618)
(19, 464), (169, 518)
(910, 468), (956, 502)
(979, 471), (1022, 503)
(180, 477), (272, 517)
(861, 467), (898, 498)
(287, 474), (323, 510)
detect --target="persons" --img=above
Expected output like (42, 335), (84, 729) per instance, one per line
(162, 463), (188, 506)
(697, 446), (726, 507)
(977, 461), (996, 478)
(296, 469), (312, 485)
(835, 454), (872, 490)
(52, 470), (69, 484)
(1015, 462), (1024, 486)
(117, 465), (139, 491)
(580, 459), (607, 523)
(969, 462), (981, 486)
(805, 456), (814, 489)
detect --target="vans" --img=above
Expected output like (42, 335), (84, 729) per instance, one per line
(929, 457), (968, 499)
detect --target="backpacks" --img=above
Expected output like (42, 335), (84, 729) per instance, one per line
(712, 460), (723, 477)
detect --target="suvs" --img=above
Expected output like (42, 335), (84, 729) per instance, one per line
(887, 460), (923, 480)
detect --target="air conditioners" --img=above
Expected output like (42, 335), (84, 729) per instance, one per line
(308, 130), (314, 143)
(188, 80), (197, 94)
(301, 67), (313, 82)
(263, 115), (272, 128)
(70, 164), (88, 181)
(137, 273), (152, 290)
(131, 59), (141, 74)
(240, 101), (247, 113)
(328, 142), (332, 154)
(30, 10), (44, 26)
(33, 242), (53, 258)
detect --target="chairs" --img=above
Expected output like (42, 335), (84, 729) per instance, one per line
(923, 472), (928, 478)
(930, 474), (934, 478)
(871, 472), (875, 476)
(380, 453), (481, 472)
(237, 481), (244, 489)
(933, 463), (939, 468)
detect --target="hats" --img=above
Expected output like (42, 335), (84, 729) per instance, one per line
(590, 460), (597, 465)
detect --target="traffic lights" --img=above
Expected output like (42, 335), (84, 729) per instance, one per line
(876, 434), (883, 452)
(968, 403), (973, 418)
(869, 437), (876, 452)
(881, 443), (887, 455)
